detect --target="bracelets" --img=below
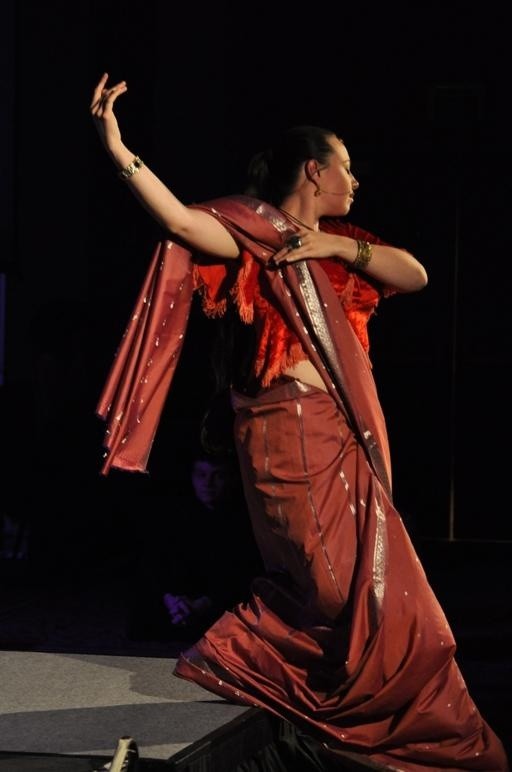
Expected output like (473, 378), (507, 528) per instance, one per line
(352, 237), (371, 272)
(119, 156), (143, 180)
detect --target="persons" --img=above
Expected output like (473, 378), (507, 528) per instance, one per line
(88, 73), (506, 770)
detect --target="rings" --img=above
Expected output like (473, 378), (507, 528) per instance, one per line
(290, 236), (302, 251)
